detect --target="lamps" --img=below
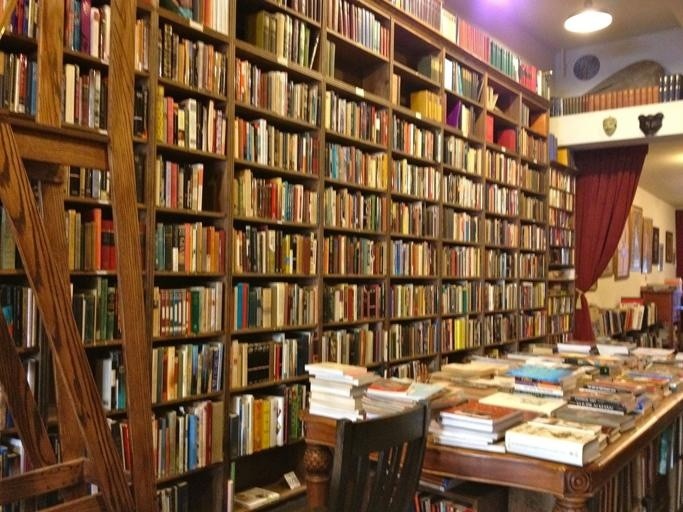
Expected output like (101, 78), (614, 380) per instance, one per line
(560, 0), (614, 36)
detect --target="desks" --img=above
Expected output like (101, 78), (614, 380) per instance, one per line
(297, 340), (683, 512)
(640, 287), (683, 350)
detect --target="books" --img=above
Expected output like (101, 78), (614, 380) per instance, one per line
(551, 330), (683, 471)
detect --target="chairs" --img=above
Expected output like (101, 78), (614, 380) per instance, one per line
(323, 404), (430, 512)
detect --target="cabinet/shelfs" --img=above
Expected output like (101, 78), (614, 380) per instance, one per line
(0, 0), (575, 512)
